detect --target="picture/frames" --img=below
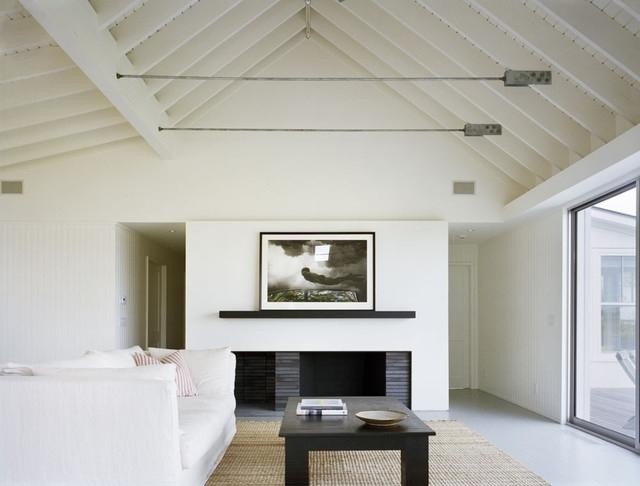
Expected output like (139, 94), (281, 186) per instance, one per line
(258, 230), (377, 313)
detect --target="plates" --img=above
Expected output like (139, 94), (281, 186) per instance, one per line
(355, 409), (409, 427)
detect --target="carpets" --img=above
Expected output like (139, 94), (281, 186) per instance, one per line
(205, 419), (552, 486)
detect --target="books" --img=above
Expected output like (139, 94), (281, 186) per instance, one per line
(301, 398), (343, 410)
(296, 403), (348, 416)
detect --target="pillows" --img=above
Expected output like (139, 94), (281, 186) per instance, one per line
(132, 349), (198, 397)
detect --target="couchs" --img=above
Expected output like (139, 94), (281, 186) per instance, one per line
(0, 344), (238, 486)
